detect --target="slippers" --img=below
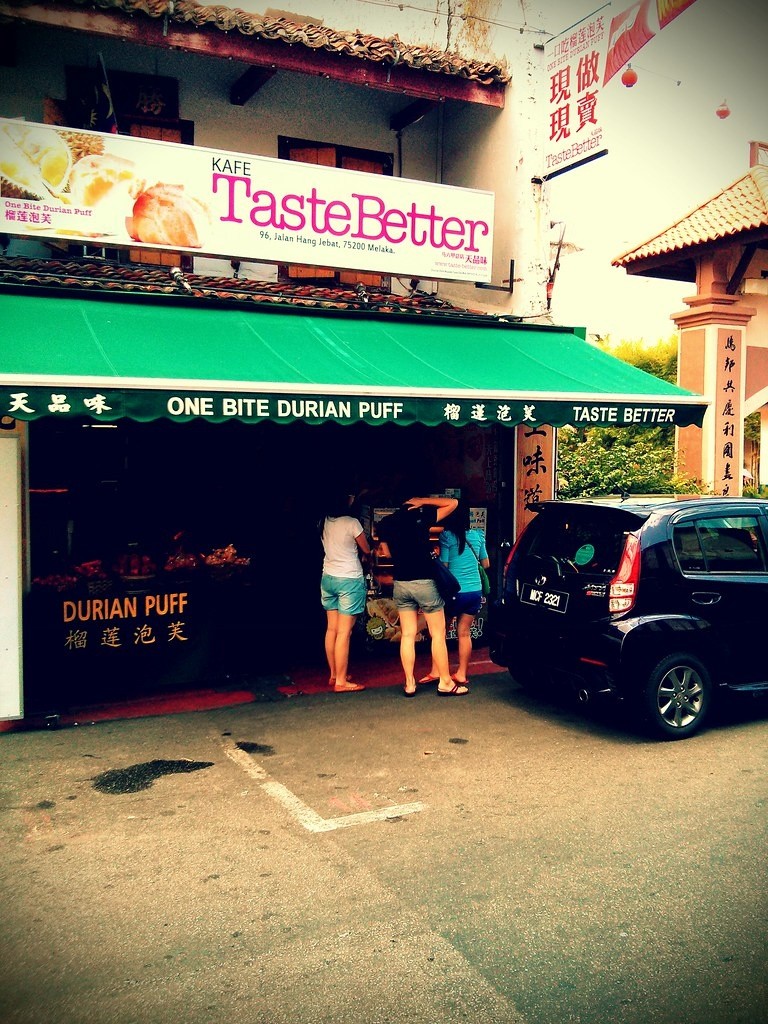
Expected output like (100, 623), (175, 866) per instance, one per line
(450, 673), (469, 684)
(403, 684), (417, 697)
(437, 686), (469, 697)
(418, 675), (440, 685)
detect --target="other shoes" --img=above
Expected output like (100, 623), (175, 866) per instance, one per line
(329, 674), (353, 685)
(334, 684), (365, 692)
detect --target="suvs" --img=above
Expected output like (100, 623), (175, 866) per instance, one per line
(490, 474), (768, 742)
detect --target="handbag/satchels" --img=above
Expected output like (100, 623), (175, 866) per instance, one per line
(479, 564), (491, 597)
(430, 550), (461, 600)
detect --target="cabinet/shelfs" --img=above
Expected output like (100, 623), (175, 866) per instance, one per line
(360, 506), (454, 605)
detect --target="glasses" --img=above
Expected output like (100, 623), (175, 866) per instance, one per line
(348, 493), (356, 498)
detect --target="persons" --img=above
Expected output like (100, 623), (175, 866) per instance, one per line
(416, 497), (491, 686)
(314, 484), (372, 693)
(359, 482), (471, 699)
